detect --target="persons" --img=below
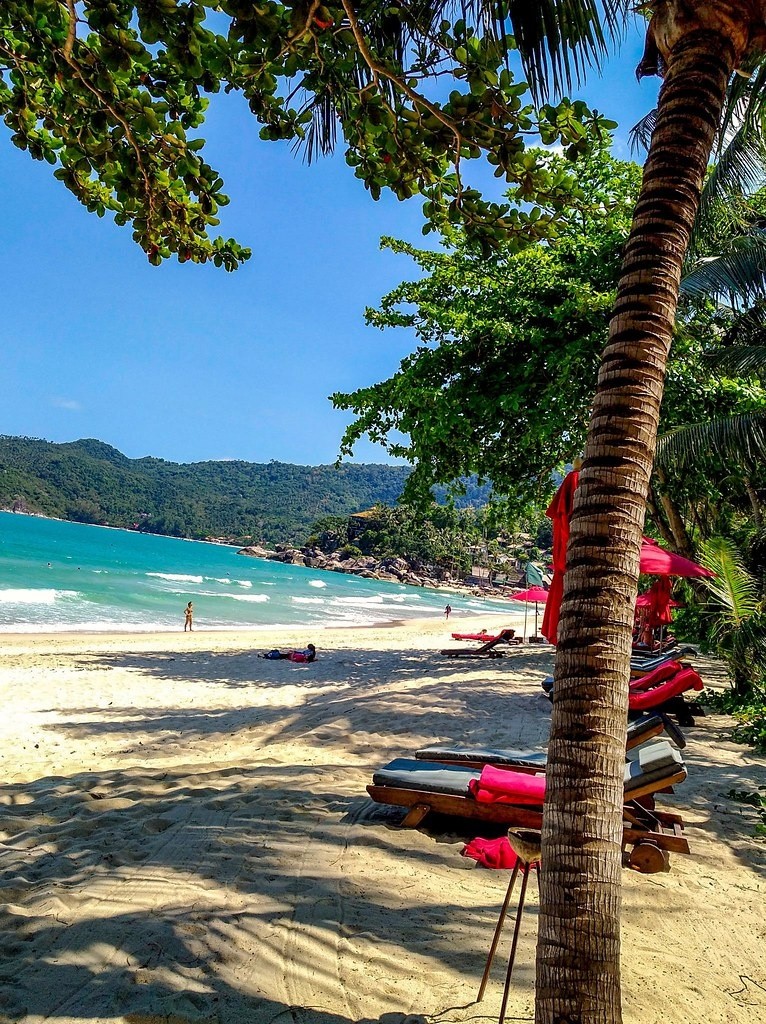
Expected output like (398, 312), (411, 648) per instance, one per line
(476, 629), (487, 635)
(290, 644), (316, 658)
(446, 604), (451, 618)
(279, 653), (313, 662)
(184, 602), (193, 631)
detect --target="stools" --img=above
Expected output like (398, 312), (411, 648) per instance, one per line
(529, 637), (543, 643)
(515, 637), (522, 642)
(508, 640), (519, 645)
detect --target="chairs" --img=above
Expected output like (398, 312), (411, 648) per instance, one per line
(542, 632), (703, 725)
(452, 632), (493, 641)
(480, 628), (515, 644)
(441, 636), (505, 659)
(366, 715), (690, 874)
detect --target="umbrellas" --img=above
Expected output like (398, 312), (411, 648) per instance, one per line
(639, 534), (717, 576)
(636, 589), (684, 606)
(541, 470), (580, 646)
(510, 587), (551, 637)
(649, 575), (672, 659)
